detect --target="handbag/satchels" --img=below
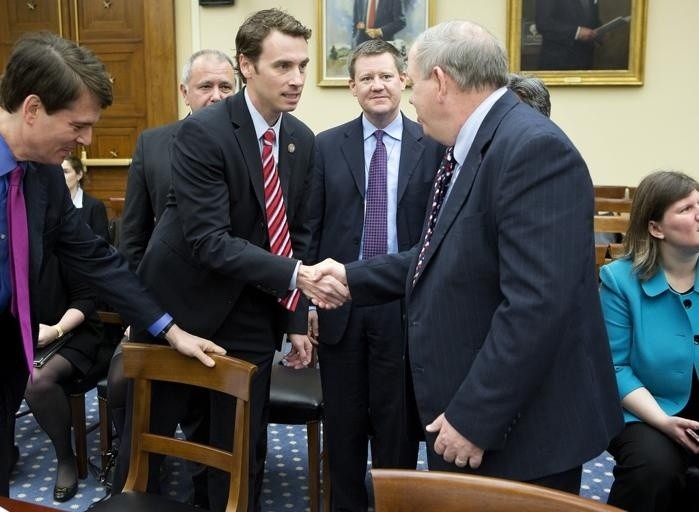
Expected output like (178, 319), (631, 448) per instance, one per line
(86, 441), (127, 490)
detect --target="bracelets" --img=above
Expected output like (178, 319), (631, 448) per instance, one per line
(158, 319), (175, 342)
(51, 320), (65, 341)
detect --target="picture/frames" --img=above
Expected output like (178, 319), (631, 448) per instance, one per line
(314, 0), (434, 91)
(506, 1), (645, 88)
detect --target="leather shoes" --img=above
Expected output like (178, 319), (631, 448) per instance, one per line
(54, 454), (80, 501)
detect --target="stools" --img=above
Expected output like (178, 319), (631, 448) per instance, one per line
(255, 358), (333, 512)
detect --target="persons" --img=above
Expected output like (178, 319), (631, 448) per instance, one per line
(599, 171), (698, 512)
(117, 51), (238, 501)
(499, 72), (553, 121)
(302, 38), (459, 510)
(59, 152), (111, 239)
(300, 20), (623, 510)
(535, 0), (606, 69)
(25, 256), (110, 502)
(144, 9), (350, 511)
(87, 315), (144, 512)
(0, 32), (226, 508)
(354, 0), (408, 56)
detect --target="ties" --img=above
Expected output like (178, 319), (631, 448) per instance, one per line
(8, 167), (33, 385)
(261, 128), (300, 312)
(413, 146), (454, 279)
(369, 0), (376, 25)
(362, 131), (388, 260)
(33, 278), (35, 279)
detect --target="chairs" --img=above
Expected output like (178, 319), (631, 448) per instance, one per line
(85, 335), (259, 511)
(368, 457), (628, 512)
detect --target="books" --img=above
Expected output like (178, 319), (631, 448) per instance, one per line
(594, 14), (631, 38)
(32, 332), (71, 368)
